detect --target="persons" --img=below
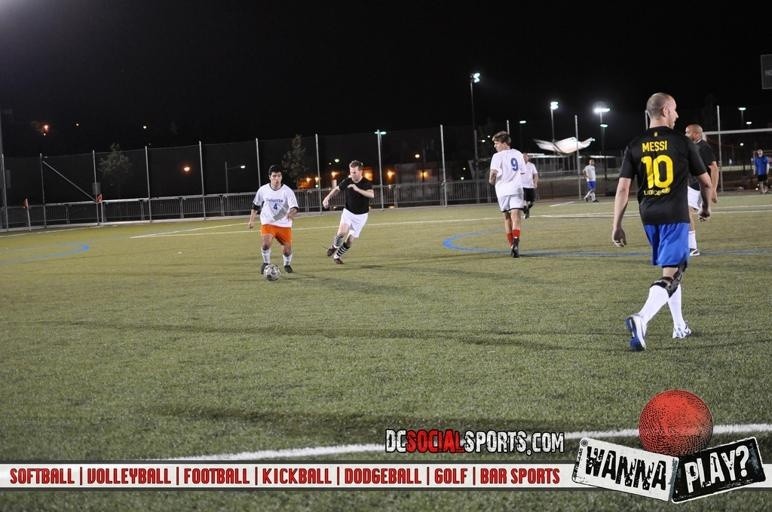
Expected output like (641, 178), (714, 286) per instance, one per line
(248, 165), (299, 276)
(488, 131), (524, 258)
(581, 159), (599, 204)
(320, 160), (375, 267)
(681, 121), (720, 257)
(520, 151), (540, 219)
(611, 90), (717, 352)
(752, 148), (770, 193)
(752, 151), (760, 191)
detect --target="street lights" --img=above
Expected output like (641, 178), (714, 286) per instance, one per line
(414, 149), (425, 196)
(223, 162), (246, 193)
(593, 106), (612, 195)
(550, 100), (560, 177)
(738, 105), (753, 171)
(374, 130), (388, 209)
(470, 72), (484, 205)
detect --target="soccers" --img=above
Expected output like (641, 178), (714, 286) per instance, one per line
(264, 264), (280, 281)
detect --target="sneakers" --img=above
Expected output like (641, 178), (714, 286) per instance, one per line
(261, 263), (268, 274)
(584, 196), (599, 202)
(673, 327), (692, 338)
(690, 248), (700, 257)
(327, 245), (336, 257)
(625, 313), (646, 351)
(335, 256), (344, 264)
(284, 265), (293, 273)
(523, 206), (530, 219)
(510, 238), (520, 258)
